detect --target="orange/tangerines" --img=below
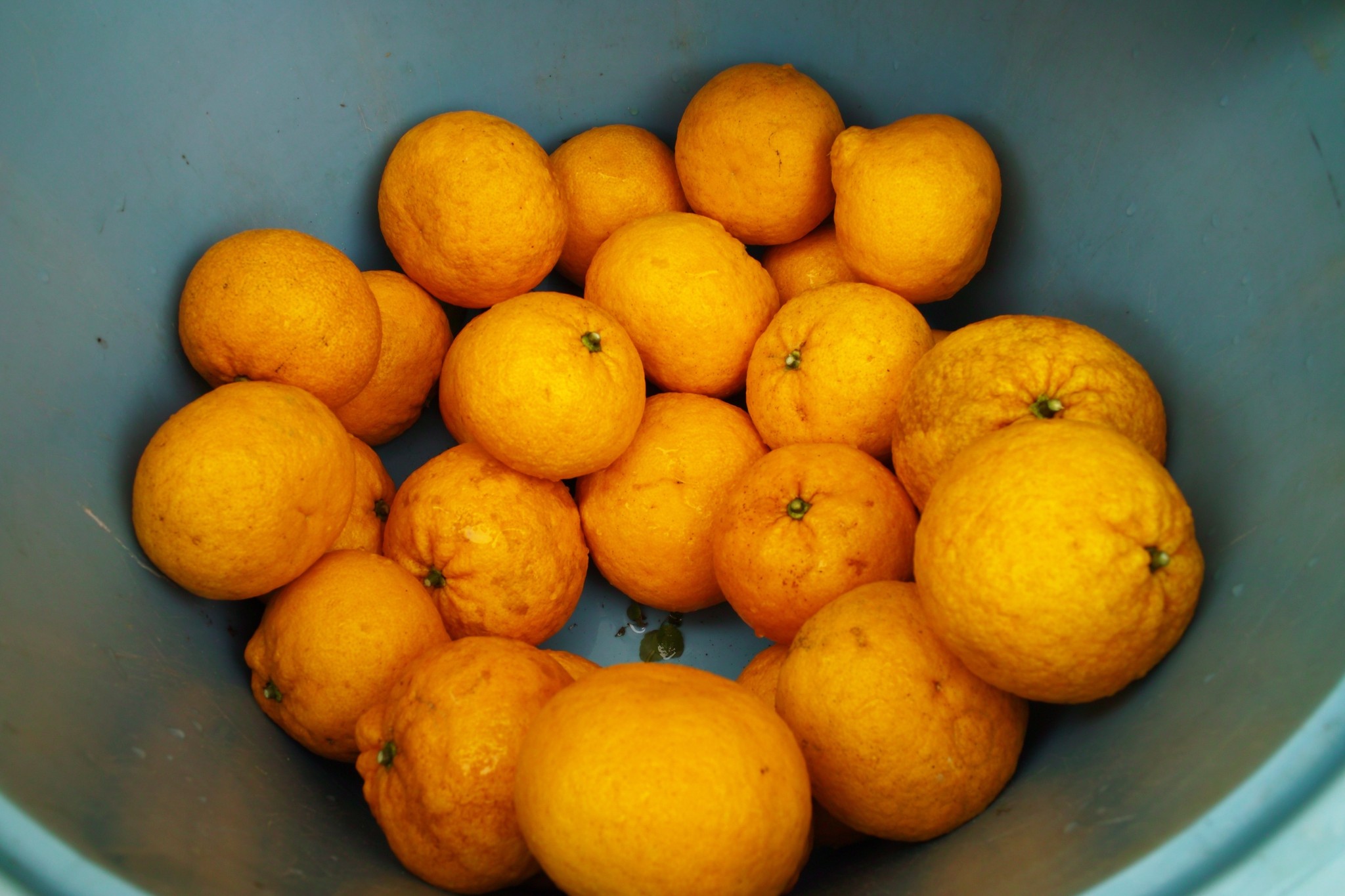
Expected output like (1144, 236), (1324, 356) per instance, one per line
(129, 62), (1202, 896)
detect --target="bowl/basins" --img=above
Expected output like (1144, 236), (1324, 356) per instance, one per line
(0, 3), (1344, 893)
